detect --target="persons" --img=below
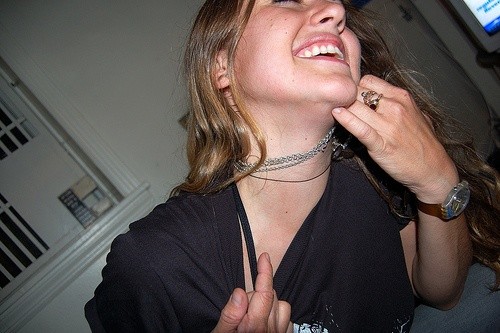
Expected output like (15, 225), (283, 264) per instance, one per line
(84, 0), (500, 333)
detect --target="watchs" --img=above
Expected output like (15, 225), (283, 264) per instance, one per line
(415, 179), (471, 221)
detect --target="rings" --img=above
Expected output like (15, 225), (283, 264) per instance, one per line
(359, 91), (384, 111)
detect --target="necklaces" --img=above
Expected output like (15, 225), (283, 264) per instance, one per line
(229, 124), (353, 183)
